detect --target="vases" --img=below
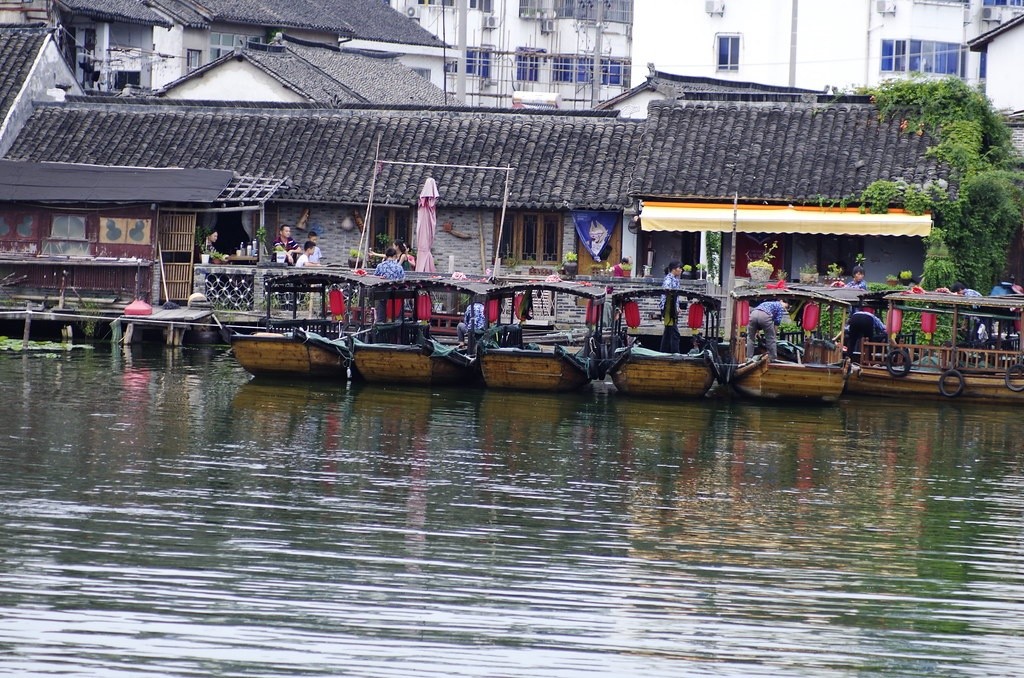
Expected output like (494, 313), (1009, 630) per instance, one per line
(749, 263), (772, 282)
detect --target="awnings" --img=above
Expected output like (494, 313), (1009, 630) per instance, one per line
(639, 205), (931, 237)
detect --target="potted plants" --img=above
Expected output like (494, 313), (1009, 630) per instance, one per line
(196, 226), (222, 264)
(433, 297), (444, 312)
(900, 269), (913, 286)
(796, 263), (820, 284)
(272, 244), (285, 262)
(886, 272), (899, 286)
(683, 263), (693, 280)
(564, 250), (579, 275)
(696, 262), (706, 279)
(823, 264), (849, 283)
(256, 227), (271, 263)
(622, 262), (632, 277)
(349, 247), (363, 268)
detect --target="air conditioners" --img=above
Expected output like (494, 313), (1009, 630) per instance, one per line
(876, 0), (897, 19)
(980, 6), (1003, 25)
(541, 21), (557, 36)
(403, 6), (422, 21)
(704, 0), (726, 17)
(481, 15), (500, 33)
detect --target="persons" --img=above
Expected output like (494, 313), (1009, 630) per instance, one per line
(373, 248), (404, 323)
(658, 260), (682, 354)
(457, 297), (485, 348)
(369, 239), (415, 271)
(747, 298), (784, 360)
(833, 311), (885, 359)
(195, 229), (218, 263)
(611, 257), (629, 277)
(271, 225), (323, 267)
(845, 266), (868, 314)
(951, 282), (983, 332)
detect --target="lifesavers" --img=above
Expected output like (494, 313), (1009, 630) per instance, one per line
(886, 349), (912, 378)
(1005, 364), (1023, 391)
(939, 370), (964, 397)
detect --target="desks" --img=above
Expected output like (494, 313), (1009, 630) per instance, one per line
(225, 251), (259, 264)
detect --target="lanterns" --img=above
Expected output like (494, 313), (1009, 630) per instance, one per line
(688, 302), (703, 334)
(386, 298), (402, 323)
(736, 301), (750, 332)
(922, 312), (936, 339)
(483, 300), (498, 323)
(886, 309), (902, 339)
(515, 293), (526, 321)
(802, 302), (818, 336)
(586, 298), (601, 324)
(625, 300), (640, 328)
(330, 289), (345, 320)
(417, 294), (431, 325)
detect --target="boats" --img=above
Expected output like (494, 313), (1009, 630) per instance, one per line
(611, 287), (722, 401)
(475, 281), (610, 397)
(341, 276), (502, 390)
(723, 284), (854, 408)
(843, 289), (1024, 408)
(229, 264), (390, 381)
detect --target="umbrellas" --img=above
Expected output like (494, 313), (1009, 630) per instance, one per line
(415, 178), (440, 272)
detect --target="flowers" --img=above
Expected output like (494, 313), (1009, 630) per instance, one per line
(743, 240), (781, 268)
